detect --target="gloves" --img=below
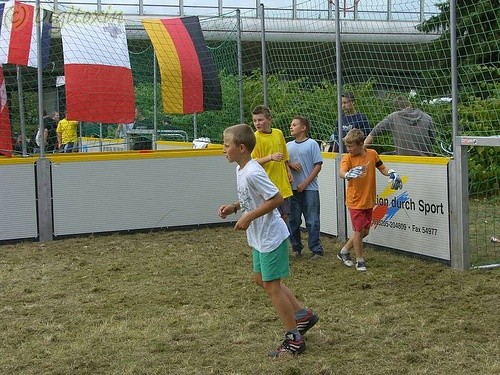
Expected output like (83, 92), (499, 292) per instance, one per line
(345, 166), (362, 181)
(388, 169), (402, 190)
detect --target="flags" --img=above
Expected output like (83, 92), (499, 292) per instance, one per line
(60, 10), (136, 123)
(0, 0), (53, 69)
(142, 16), (223, 114)
(0, 64), (12, 157)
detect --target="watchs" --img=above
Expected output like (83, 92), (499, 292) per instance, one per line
(232, 204), (237, 214)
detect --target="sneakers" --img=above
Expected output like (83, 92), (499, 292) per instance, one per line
(311, 255), (323, 260)
(296, 312), (320, 335)
(356, 257), (366, 272)
(268, 333), (307, 358)
(292, 251), (300, 257)
(337, 250), (354, 266)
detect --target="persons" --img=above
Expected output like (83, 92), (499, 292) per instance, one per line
(16, 134), (30, 153)
(336, 128), (403, 272)
(217, 123), (319, 356)
(56, 111), (79, 153)
(332, 91), (372, 152)
(44, 110), (59, 154)
(286, 117), (325, 258)
(250, 105), (293, 226)
(364, 96), (437, 156)
